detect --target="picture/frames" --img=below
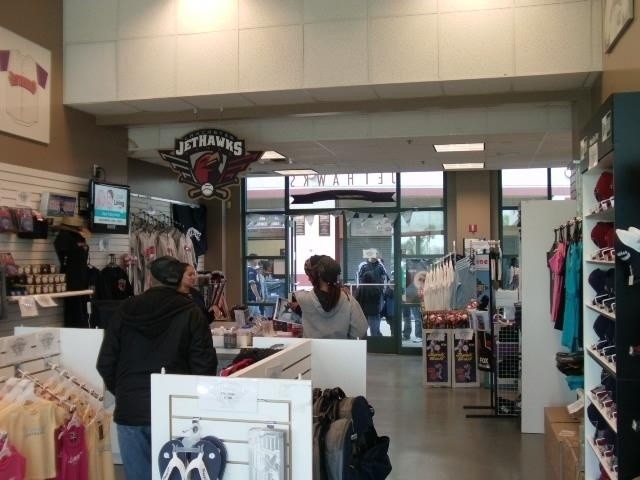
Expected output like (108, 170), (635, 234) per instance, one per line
(604, 1), (634, 55)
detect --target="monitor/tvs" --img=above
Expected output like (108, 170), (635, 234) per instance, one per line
(88, 178), (131, 235)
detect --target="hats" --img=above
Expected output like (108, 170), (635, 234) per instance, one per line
(147, 255), (184, 286)
(51, 215), (92, 239)
(313, 256), (341, 274)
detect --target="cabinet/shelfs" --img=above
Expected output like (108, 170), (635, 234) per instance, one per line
(583, 90), (640, 480)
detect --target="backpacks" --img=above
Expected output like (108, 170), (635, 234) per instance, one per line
(313, 387), (393, 480)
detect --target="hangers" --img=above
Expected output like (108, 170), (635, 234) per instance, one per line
(0, 432), (14, 459)
(426, 239), (458, 277)
(0, 358), (111, 440)
(554, 216), (582, 247)
(170, 423), (203, 454)
(129, 208), (187, 238)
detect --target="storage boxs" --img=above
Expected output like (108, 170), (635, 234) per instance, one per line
(563, 436), (584, 480)
(548, 422), (584, 480)
(544, 406), (583, 459)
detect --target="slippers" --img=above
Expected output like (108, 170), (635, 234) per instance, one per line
(186, 439), (223, 480)
(158, 439), (186, 480)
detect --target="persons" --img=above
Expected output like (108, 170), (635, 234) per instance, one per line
(97, 256), (218, 480)
(292, 255), (368, 339)
(257, 260), (272, 316)
(358, 248), (389, 283)
(384, 277), (399, 337)
(400, 271), (424, 342)
(178, 263), (221, 323)
(104, 189), (120, 211)
(247, 253), (262, 302)
(352, 273), (383, 336)
